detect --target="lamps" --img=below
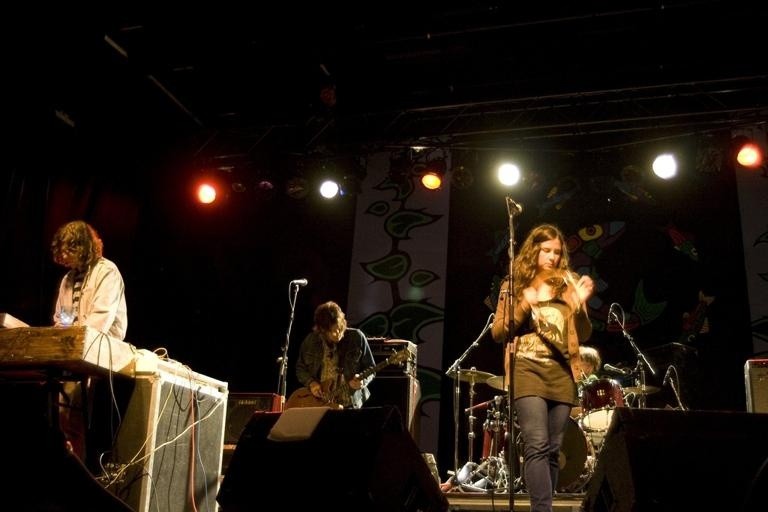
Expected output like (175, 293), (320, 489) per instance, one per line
(419, 148), (448, 190)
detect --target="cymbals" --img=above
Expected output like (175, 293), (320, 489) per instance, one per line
(449, 369), (496, 384)
(624, 386), (660, 395)
(486, 376), (511, 394)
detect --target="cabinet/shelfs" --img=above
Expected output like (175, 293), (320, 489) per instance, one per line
(362, 375), (422, 431)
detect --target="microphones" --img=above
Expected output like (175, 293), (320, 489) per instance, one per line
(607, 303), (616, 324)
(603, 363), (626, 375)
(662, 368), (672, 385)
(292, 277), (308, 287)
(507, 196), (523, 215)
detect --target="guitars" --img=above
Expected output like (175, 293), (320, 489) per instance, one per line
(284, 348), (412, 408)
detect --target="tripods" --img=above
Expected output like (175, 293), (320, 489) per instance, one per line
(463, 398), (509, 495)
(457, 373), (488, 485)
(444, 327), (494, 493)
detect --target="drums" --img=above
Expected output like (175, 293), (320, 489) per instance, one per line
(504, 415), (588, 491)
(580, 379), (624, 432)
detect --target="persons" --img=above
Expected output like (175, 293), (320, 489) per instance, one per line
(51, 220), (127, 459)
(572, 345), (599, 393)
(294, 300), (377, 411)
(491, 222), (593, 512)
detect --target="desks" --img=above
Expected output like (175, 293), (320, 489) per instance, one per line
(216, 410), (451, 512)
(577, 407), (767, 512)
